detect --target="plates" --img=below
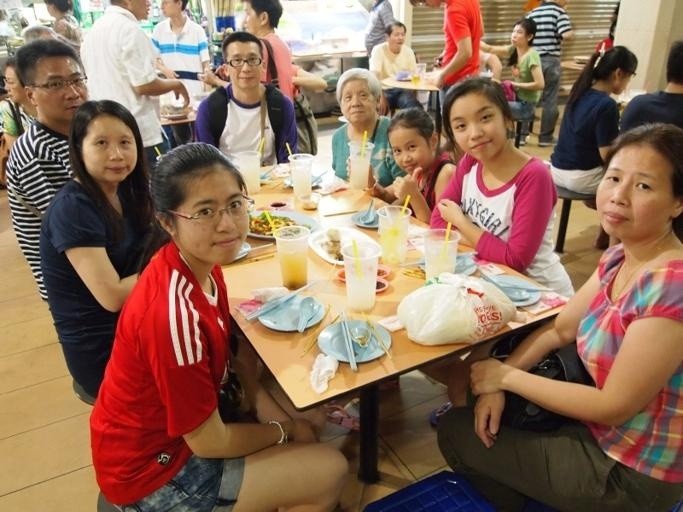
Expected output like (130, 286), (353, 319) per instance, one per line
(246, 209), (318, 239)
(257, 293), (325, 332)
(479, 275), (541, 307)
(159, 106), (196, 122)
(306, 227), (383, 266)
(375, 278), (389, 294)
(284, 175), (322, 187)
(234, 241), (252, 262)
(336, 268), (348, 283)
(419, 252), (477, 277)
(376, 263), (391, 279)
(317, 319), (391, 364)
(350, 211), (380, 229)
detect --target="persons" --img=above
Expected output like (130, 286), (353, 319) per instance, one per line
(344, 107), (458, 225)
(477, 21), (544, 121)
(412, 1), (484, 88)
(362, 0), (396, 56)
(332, 68), (407, 198)
(551, 46), (638, 247)
(621, 40), (683, 133)
(78, 0), (191, 168)
(478, 40), (503, 84)
(198, 0), (294, 109)
(518, 1), (576, 146)
(417, 77), (575, 427)
(24, 26), (59, 45)
(195, 32), (297, 166)
(40, 0), (81, 46)
(90, 143), (346, 510)
(39, 99), (272, 397)
(0, 62), (37, 154)
(8, 39), (89, 299)
(369, 23), (425, 111)
(152, 0), (213, 148)
(291, 61), (327, 155)
(437, 123), (683, 511)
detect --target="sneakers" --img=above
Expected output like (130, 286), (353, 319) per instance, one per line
(538, 137), (560, 147)
(519, 135), (530, 146)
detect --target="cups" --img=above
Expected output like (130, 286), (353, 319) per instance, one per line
(347, 140), (374, 189)
(340, 243), (381, 311)
(273, 226), (310, 291)
(377, 206), (412, 265)
(287, 153), (313, 197)
(269, 201), (289, 215)
(423, 228), (461, 281)
(238, 150), (261, 195)
(300, 193), (322, 211)
(170, 91), (188, 109)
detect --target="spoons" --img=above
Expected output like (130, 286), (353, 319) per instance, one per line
(477, 266), (530, 302)
(296, 296), (318, 334)
(350, 327), (371, 348)
(319, 179), (346, 196)
(359, 199), (374, 224)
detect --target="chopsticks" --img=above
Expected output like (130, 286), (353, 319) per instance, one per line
(338, 310), (358, 373)
(323, 209), (358, 217)
(311, 169), (328, 185)
(244, 282), (313, 322)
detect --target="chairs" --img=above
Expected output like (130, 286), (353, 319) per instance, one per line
(341, 56), (399, 119)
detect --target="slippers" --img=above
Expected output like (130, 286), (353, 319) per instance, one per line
(430, 401), (456, 428)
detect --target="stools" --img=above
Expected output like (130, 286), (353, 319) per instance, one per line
(512, 114), (535, 148)
(362, 470), (497, 512)
(553, 185), (609, 254)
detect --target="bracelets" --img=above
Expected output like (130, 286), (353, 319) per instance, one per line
(270, 420), (288, 446)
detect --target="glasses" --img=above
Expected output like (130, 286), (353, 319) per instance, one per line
(227, 58), (263, 67)
(31, 75), (89, 92)
(622, 70), (637, 80)
(159, 195), (255, 224)
(4, 79), (25, 87)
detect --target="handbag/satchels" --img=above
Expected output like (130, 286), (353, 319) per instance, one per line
(290, 95), (319, 156)
(260, 36), (282, 90)
(490, 335), (592, 431)
(0, 100), (26, 185)
